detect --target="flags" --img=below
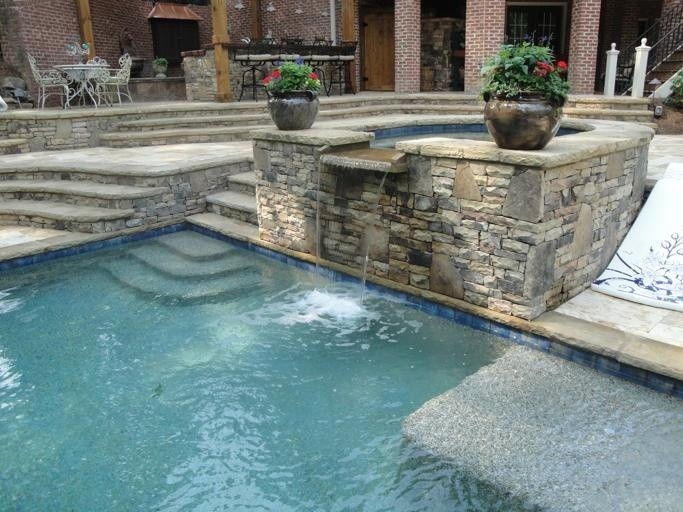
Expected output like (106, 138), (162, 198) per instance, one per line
(265, 89), (320, 131)
(482, 91), (565, 151)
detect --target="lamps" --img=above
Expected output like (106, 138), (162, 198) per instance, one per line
(27, 52), (132, 108)
(231, 34), (358, 103)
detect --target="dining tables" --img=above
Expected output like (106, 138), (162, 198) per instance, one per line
(152, 55), (168, 78)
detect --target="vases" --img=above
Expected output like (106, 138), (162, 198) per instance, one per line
(477, 27), (574, 108)
